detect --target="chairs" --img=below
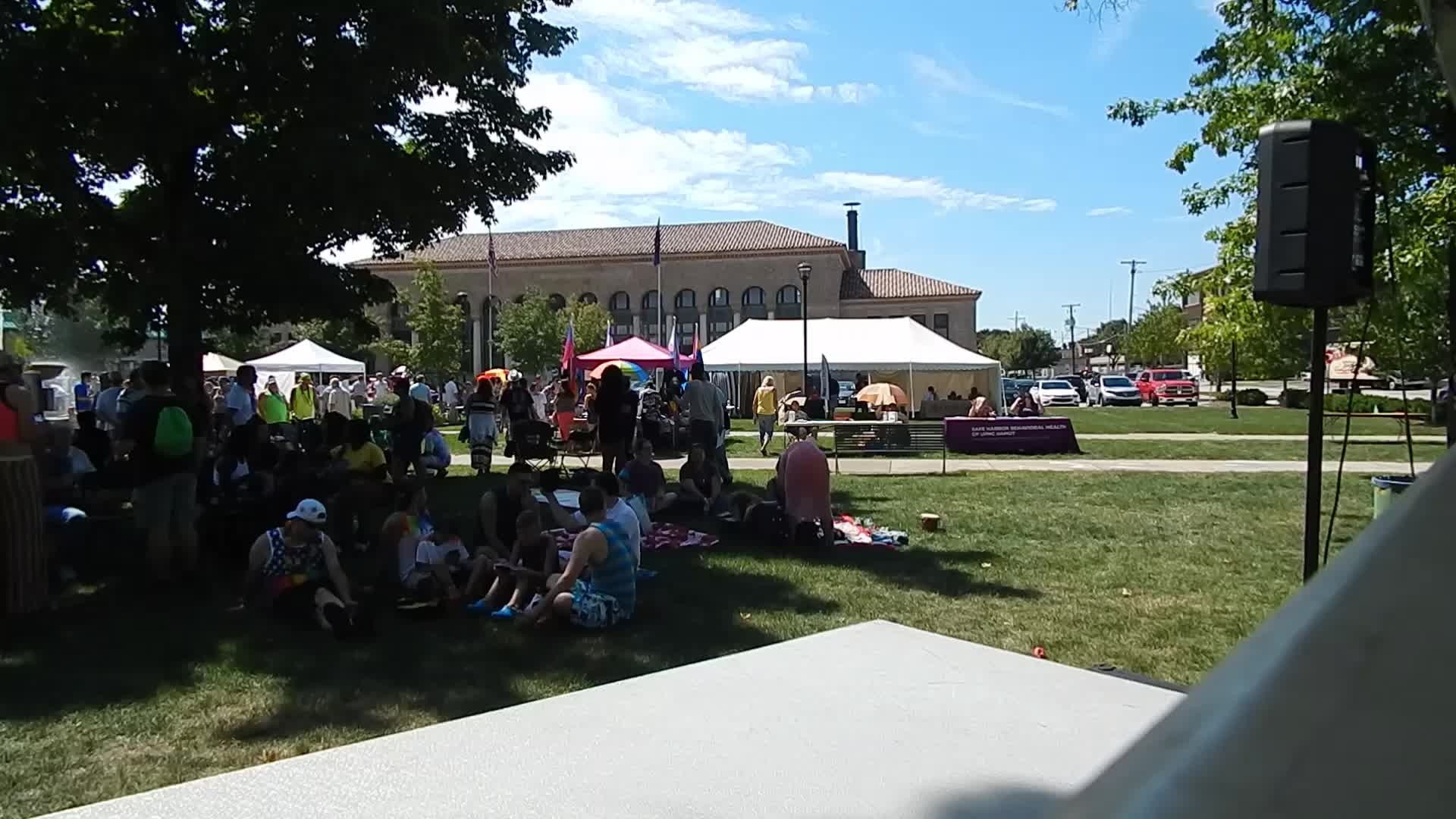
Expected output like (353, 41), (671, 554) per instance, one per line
(558, 430), (598, 479)
(784, 406), (810, 446)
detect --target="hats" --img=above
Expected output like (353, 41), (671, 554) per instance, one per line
(286, 498), (327, 522)
(508, 369), (522, 381)
(298, 373), (313, 384)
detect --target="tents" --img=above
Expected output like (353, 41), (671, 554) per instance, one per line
(568, 337), (695, 391)
(690, 316), (1008, 418)
(203, 352), (244, 376)
(245, 338), (366, 412)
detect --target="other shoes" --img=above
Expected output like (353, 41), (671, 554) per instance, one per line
(492, 607), (522, 618)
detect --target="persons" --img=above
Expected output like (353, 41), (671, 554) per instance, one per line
(947, 391), (961, 400)
(925, 386), (939, 401)
(1009, 393), (1045, 417)
(879, 403), (907, 423)
(829, 378), (840, 418)
(968, 387), (997, 417)
(0, 350), (834, 649)
(853, 374), (868, 413)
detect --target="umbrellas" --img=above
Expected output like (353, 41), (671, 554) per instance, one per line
(856, 383), (906, 411)
(475, 368), (510, 382)
(776, 389), (808, 427)
(588, 360), (649, 382)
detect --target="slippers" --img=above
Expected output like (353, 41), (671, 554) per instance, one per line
(468, 600), (497, 613)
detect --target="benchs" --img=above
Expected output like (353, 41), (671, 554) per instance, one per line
(833, 424), (947, 476)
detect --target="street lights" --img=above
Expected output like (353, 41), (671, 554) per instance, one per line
(796, 259), (813, 397)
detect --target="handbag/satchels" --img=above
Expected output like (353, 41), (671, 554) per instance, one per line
(458, 426), (469, 443)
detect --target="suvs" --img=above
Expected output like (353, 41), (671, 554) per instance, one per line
(1134, 369), (1198, 408)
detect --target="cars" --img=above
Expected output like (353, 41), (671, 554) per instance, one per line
(1028, 380), (1080, 407)
(1053, 374), (1086, 402)
(1086, 374), (1141, 407)
(837, 380), (857, 407)
(1000, 377), (1035, 407)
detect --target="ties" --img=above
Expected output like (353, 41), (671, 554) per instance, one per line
(249, 392), (255, 413)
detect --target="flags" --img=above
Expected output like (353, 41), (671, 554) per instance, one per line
(562, 324), (574, 369)
(487, 234), (498, 278)
(668, 317), (680, 370)
(605, 321), (614, 348)
(654, 219), (660, 267)
(693, 325), (703, 363)
(821, 355), (831, 401)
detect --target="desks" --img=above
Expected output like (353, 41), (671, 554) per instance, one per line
(944, 417), (1081, 455)
(921, 400), (971, 418)
(784, 421), (849, 454)
